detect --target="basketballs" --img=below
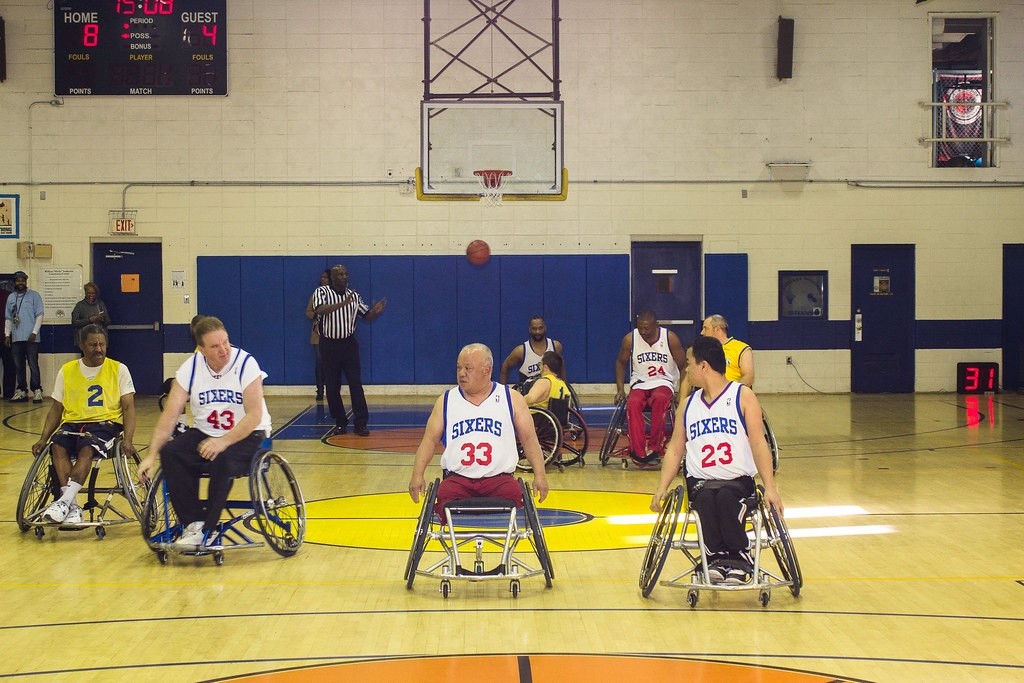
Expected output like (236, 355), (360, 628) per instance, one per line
(467, 240), (490, 266)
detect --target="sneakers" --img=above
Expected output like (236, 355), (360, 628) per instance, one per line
(62, 504), (84, 523)
(10, 389), (28, 402)
(33, 389), (43, 403)
(708, 565), (730, 583)
(42, 500), (70, 523)
(725, 568), (754, 585)
(175, 521), (220, 546)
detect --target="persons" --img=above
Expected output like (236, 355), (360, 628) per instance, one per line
(312, 265), (386, 436)
(499, 315), (566, 395)
(137, 315), (271, 546)
(72, 282), (110, 359)
(0, 271), (43, 404)
(675, 314), (755, 416)
(615, 310), (688, 468)
(409, 343), (549, 525)
(522, 351), (570, 418)
(305, 269), (331, 401)
(30, 324), (137, 524)
(650, 336), (784, 586)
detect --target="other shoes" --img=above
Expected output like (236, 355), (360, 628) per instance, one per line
(647, 450), (658, 464)
(355, 425), (369, 436)
(334, 424), (346, 434)
(630, 453), (645, 465)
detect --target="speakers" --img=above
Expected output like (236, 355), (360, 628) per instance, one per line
(776, 18), (794, 78)
(0, 18), (6, 81)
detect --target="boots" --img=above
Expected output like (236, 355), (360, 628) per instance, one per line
(316, 387), (324, 400)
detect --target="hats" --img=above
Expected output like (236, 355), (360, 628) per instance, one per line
(13, 271), (28, 280)
